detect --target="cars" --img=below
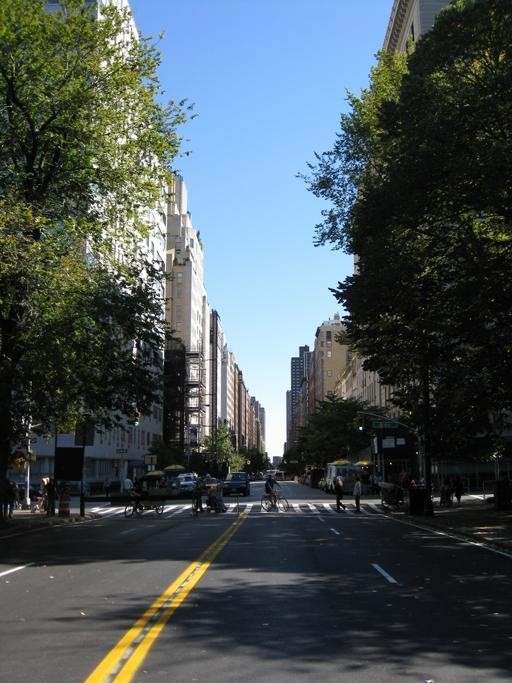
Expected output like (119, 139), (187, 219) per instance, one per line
(331, 477), (368, 496)
(172, 473), (217, 496)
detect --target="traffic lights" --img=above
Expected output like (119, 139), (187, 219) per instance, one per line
(130, 402), (140, 426)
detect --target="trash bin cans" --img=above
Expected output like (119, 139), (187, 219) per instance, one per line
(410, 486), (432, 516)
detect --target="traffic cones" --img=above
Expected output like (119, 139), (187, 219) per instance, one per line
(59, 489), (70, 517)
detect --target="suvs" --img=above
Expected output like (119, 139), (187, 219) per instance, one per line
(222, 472), (250, 496)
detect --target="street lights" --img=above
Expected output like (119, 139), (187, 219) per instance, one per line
(356, 411), (422, 481)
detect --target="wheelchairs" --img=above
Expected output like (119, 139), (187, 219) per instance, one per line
(206, 496), (227, 513)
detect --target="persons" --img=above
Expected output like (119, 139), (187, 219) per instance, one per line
(103, 477), (113, 497)
(192, 477), (227, 512)
(454, 474), (464, 504)
(333, 468), (425, 511)
(265, 475), (281, 506)
(3, 478), (56, 519)
(120, 475), (134, 497)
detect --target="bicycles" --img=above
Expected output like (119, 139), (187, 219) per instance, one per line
(382, 488), (399, 510)
(124, 478), (166, 517)
(190, 472), (202, 511)
(261, 489), (289, 512)
